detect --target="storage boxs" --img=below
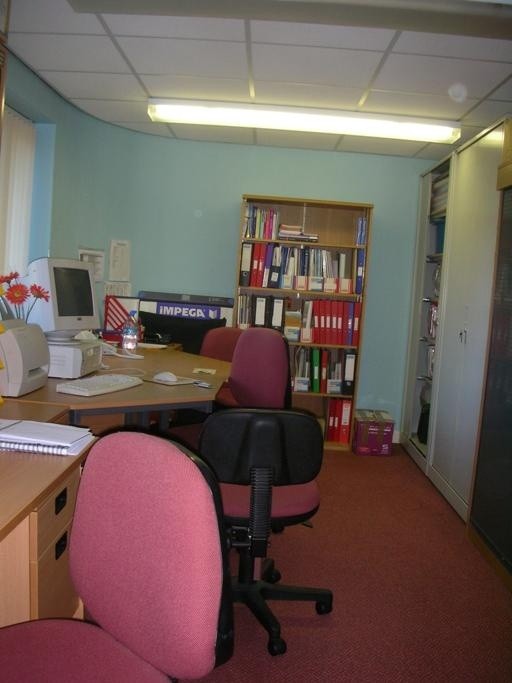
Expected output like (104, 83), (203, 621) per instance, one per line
(352, 407), (396, 458)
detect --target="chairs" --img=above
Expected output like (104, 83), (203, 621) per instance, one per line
(166, 327), (245, 448)
(227, 324), (293, 553)
(0, 422), (237, 683)
(200, 406), (334, 658)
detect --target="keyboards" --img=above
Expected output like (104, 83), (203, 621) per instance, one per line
(57, 373), (144, 395)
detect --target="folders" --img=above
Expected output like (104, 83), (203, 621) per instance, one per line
(310, 347), (356, 395)
(239, 243), (274, 288)
(325, 398), (353, 443)
(253, 295), (284, 331)
(357, 249), (364, 294)
(312, 300), (362, 347)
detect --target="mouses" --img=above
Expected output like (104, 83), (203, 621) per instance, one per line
(154, 370), (178, 383)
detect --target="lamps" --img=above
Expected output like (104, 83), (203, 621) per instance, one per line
(145, 94), (462, 144)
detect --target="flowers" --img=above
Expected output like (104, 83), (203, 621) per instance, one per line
(0, 269), (50, 322)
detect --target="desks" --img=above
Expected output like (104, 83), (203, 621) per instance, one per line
(0, 340), (232, 438)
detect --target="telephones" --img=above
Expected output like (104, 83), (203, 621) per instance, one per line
(101, 342), (117, 356)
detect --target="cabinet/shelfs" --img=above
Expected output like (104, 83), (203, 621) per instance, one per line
(400, 153), (455, 476)
(231, 193), (374, 451)
(1, 400), (99, 631)
(426, 119), (505, 527)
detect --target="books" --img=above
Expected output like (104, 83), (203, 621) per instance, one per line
(238, 204), (367, 445)
(0, 419), (95, 456)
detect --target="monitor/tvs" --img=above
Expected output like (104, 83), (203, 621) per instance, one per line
(9, 257), (102, 344)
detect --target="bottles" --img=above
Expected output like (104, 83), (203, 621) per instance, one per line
(122, 310), (138, 353)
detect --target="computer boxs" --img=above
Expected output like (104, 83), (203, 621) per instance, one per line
(47, 342), (105, 379)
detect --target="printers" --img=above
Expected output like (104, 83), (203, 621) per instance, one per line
(1, 318), (53, 397)
